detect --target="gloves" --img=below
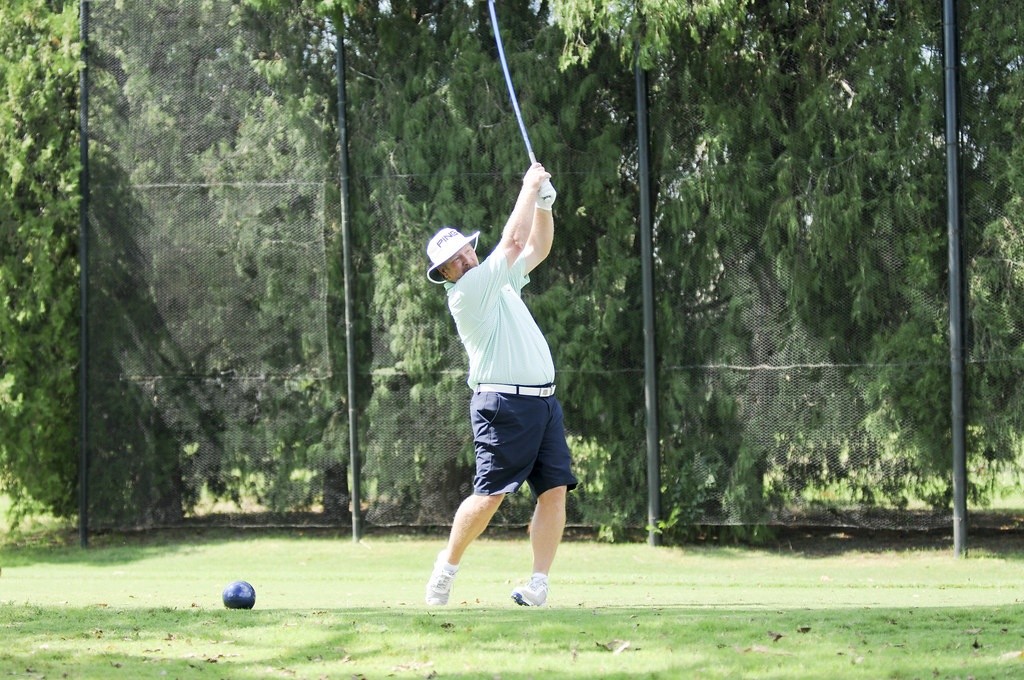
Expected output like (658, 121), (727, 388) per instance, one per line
(534, 178), (557, 210)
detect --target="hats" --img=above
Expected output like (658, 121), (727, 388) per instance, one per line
(426, 227), (481, 284)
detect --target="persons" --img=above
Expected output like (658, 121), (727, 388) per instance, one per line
(425, 161), (579, 607)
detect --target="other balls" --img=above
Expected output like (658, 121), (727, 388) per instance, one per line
(221, 581), (256, 610)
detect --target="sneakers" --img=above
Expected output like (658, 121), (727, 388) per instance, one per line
(423, 549), (459, 605)
(509, 577), (549, 607)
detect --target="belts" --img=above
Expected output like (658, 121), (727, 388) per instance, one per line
(479, 383), (557, 397)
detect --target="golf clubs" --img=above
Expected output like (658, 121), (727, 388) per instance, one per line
(488, 0), (554, 205)
